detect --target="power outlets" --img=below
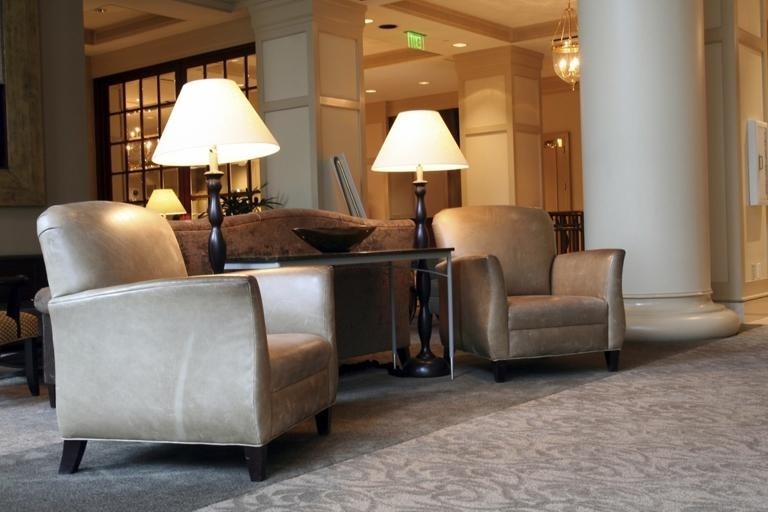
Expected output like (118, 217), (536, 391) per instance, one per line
(753, 263), (761, 278)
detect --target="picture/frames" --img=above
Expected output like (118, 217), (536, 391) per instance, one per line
(0, 1), (46, 209)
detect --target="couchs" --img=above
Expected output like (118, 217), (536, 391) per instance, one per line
(167, 209), (417, 374)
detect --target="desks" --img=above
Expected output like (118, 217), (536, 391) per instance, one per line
(223, 243), (457, 381)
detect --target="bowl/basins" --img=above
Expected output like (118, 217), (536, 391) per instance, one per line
(290, 225), (377, 252)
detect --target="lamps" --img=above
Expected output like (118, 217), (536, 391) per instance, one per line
(153, 78), (279, 274)
(143, 187), (186, 218)
(371, 109), (468, 378)
(550, 3), (579, 90)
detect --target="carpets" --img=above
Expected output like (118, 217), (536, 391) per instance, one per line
(0, 318), (768, 512)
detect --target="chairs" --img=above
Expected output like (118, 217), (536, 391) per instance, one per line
(0, 272), (43, 397)
(34, 286), (56, 409)
(430, 205), (627, 383)
(35, 202), (341, 481)
